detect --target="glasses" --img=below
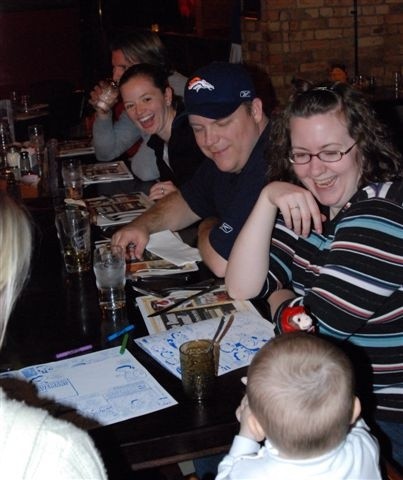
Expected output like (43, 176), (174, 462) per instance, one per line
(289, 141), (356, 164)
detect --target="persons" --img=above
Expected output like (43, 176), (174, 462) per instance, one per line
(225, 79), (403, 473)
(0, 193), (105, 480)
(214, 332), (383, 480)
(119, 64), (204, 247)
(110, 64), (290, 323)
(88, 30), (188, 182)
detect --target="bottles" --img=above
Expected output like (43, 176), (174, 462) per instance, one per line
(0, 120), (57, 175)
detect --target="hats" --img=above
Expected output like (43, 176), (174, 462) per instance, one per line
(177, 61), (256, 120)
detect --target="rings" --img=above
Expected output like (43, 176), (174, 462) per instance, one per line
(290, 206), (300, 209)
(162, 190), (163, 192)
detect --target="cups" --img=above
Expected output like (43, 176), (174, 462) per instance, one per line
(93, 245), (127, 291)
(10, 87), (19, 105)
(179, 340), (220, 404)
(21, 96), (31, 113)
(392, 71), (401, 91)
(94, 80), (120, 114)
(60, 158), (83, 200)
(55, 210), (92, 267)
(99, 290), (127, 322)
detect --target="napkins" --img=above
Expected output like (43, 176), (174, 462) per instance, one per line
(145, 229), (202, 266)
(59, 147), (96, 157)
(97, 209), (147, 226)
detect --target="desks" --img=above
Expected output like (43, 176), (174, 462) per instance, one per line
(366, 85), (403, 107)
(0, 135), (276, 480)
